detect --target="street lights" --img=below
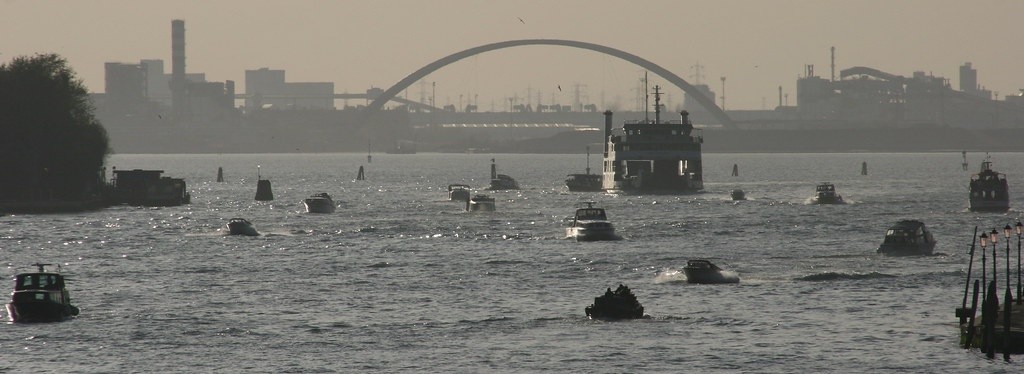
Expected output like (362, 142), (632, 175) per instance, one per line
(1015, 220), (1022, 305)
(1003, 223), (1013, 292)
(978, 230), (989, 305)
(990, 228), (999, 295)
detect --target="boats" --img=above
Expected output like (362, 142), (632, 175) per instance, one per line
(300, 192), (337, 214)
(5, 262), (79, 324)
(967, 150), (1011, 212)
(563, 145), (603, 193)
(486, 157), (521, 190)
(729, 188), (745, 201)
(600, 69), (705, 197)
(584, 283), (644, 317)
(680, 255), (724, 285)
(565, 200), (616, 242)
(875, 218), (937, 257)
(226, 217), (260, 237)
(467, 193), (496, 213)
(254, 164), (274, 202)
(816, 179), (844, 204)
(446, 182), (473, 202)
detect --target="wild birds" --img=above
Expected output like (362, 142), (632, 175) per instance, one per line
(558, 85), (562, 91)
(158, 114), (162, 119)
(516, 17), (525, 24)
